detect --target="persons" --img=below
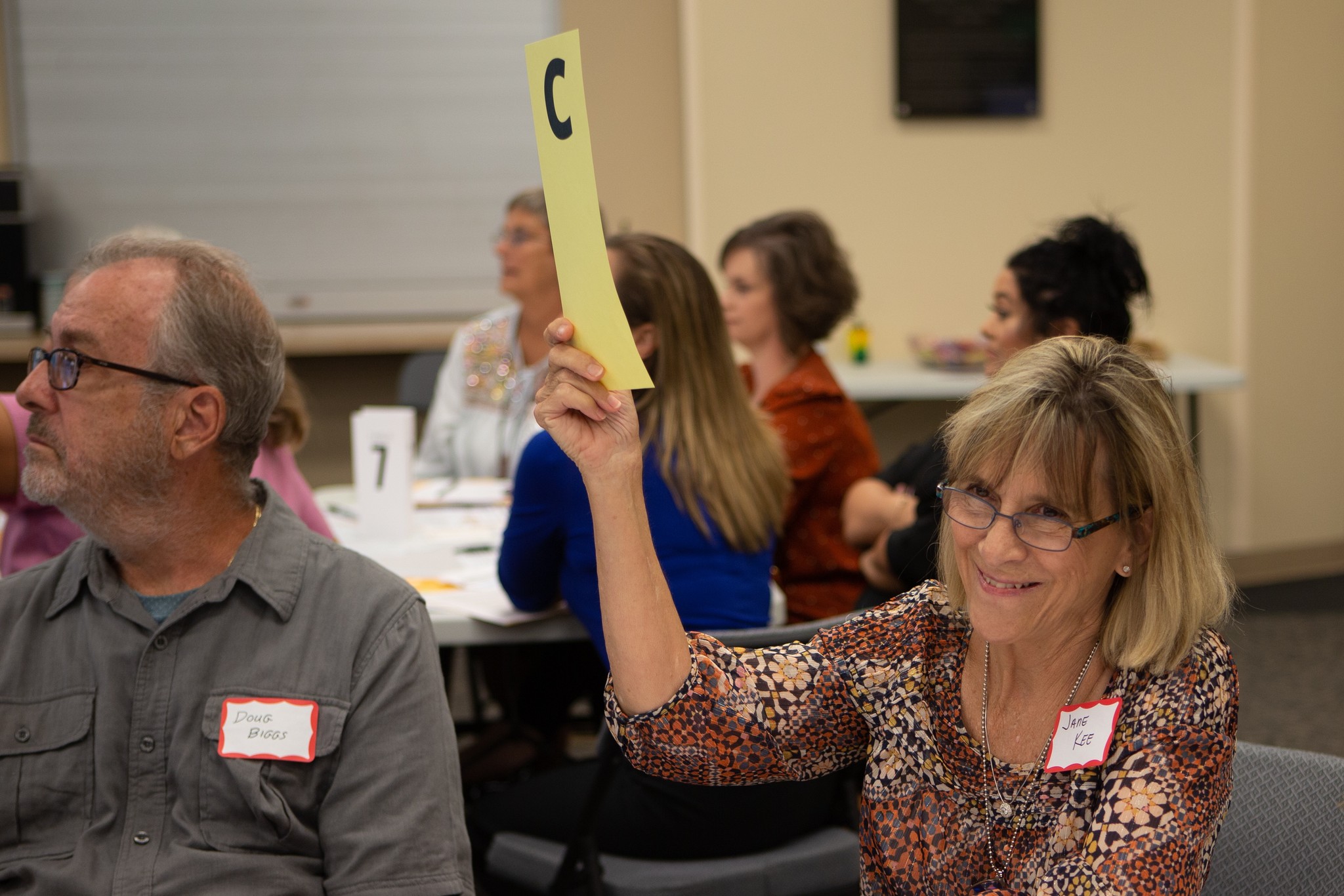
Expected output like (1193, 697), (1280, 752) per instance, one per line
(414, 190), (608, 760)
(0, 358), (337, 577)
(718, 210), (881, 628)
(468, 231), (851, 858)
(841, 216), (1152, 615)
(0, 228), (478, 896)
(534, 316), (1244, 896)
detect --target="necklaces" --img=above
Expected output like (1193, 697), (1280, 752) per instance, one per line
(227, 498), (263, 570)
(979, 636), (1097, 884)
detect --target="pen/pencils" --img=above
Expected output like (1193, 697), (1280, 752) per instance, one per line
(327, 504), (355, 518)
(455, 546), (496, 554)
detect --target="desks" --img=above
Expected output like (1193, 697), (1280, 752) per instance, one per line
(833, 358), (1245, 469)
(1, 313), (471, 367)
(317, 480), (590, 759)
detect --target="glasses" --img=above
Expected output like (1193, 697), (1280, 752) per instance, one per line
(26, 344), (200, 391)
(934, 471), (1153, 552)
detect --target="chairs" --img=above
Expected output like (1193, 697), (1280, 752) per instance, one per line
(479, 607), (1344, 896)
